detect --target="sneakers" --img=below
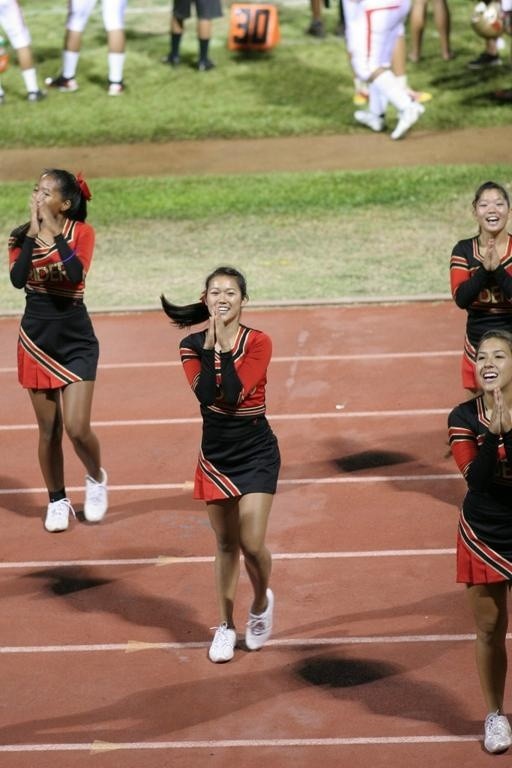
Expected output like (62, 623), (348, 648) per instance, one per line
(27, 74), (76, 100)
(209, 622), (237, 663)
(468, 52), (502, 70)
(245, 588), (274, 650)
(45, 497), (76, 532)
(107, 80), (125, 95)
(484, 709), (512, 754)
(354, 92), (433, 139)
(83, 468), (109, 522)
(308, 22), (345, 35)
(161, 54), (212, 70)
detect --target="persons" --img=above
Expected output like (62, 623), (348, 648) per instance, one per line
(348, 15), (433, 105)
(8, 168), (107, 531)
(341, 1), (426, 140)
(160, 1), (224, 71)
(446, 327), (512, 753)
(158, 267), (280, 665)
(44, 1), (131, 95)
(406, 1), (455, 63)
(306, 0), (344, 36)
(0, 1), (47, 103)
(450, 180), (511, 397)
